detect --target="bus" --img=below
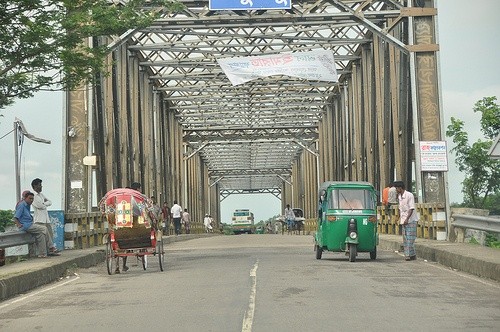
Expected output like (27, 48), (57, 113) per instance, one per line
(231, 209), (254, 234)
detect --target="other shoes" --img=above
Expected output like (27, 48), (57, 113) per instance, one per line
(115, 269), (119, 273)
(49, 253), (59, 256)
(406, 256), (415, 261)
(49, 248), (57, 252)
(123, 267), (128, 271)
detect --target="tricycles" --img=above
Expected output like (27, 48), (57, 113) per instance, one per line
(97, 188), (166, 275)
(280, 208), (306, 236)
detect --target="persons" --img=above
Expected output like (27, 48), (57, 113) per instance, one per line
(383, 183), (398, 216)
(204, 214), (214, 233)
(182, 209), (191, 234)
(170, 201), (183, 235)
(13, 190), (63, 256)
(150, 196), (160, 210)
(115, 187), (130, 274)
(394, 181), (417, 260)
(31, 178), (61, 254)
(161, 201), (172, 235)
(131, 182), (141, 191)
(285, 204), (295, 229)
(266, 222), (278, 234)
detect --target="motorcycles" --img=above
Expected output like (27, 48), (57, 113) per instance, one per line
(313, 180), (379, 263)
